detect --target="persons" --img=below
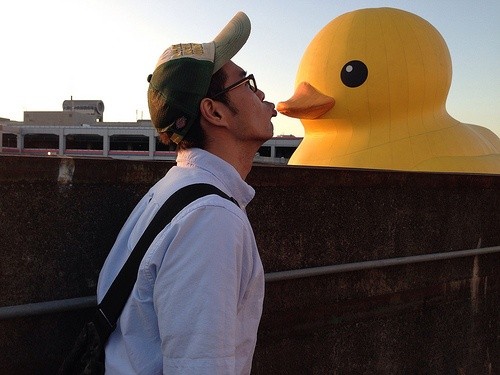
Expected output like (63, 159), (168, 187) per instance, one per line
(96, 12), (277, 375)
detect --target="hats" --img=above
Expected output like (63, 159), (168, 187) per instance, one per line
(146, 12), (252, 132)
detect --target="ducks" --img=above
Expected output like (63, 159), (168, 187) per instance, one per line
(276, 6), (499, 173)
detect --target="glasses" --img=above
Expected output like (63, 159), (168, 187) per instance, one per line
(213, 74), (257, 101)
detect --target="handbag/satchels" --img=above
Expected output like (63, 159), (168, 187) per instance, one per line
(57, 321), (106, 375)
